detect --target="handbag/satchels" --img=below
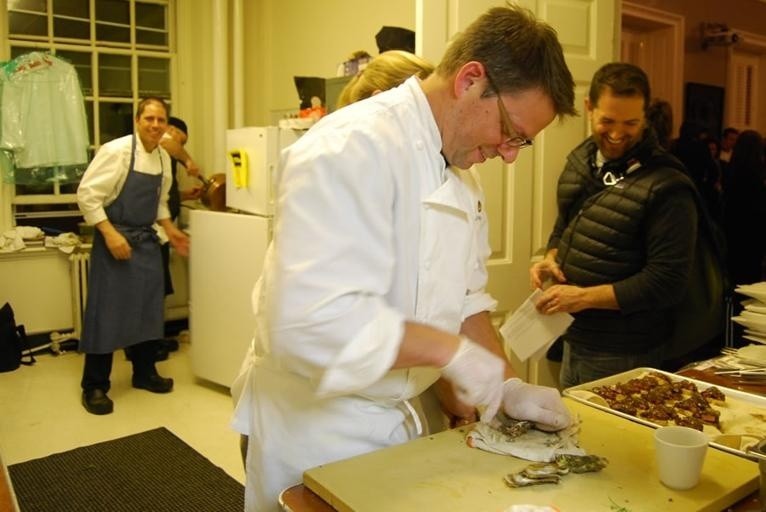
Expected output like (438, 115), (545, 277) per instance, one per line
(658, 231), (729, 360)
(0, 302), (35, 372)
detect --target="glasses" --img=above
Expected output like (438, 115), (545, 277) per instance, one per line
(488, 75), (533, 149)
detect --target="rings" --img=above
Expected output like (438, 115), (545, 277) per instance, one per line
(462, 420), (469, 424)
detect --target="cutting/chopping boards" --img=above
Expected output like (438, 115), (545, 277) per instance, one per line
(303, 396), (762, 512)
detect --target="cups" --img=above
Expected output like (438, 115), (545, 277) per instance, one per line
(655, 424), (709, 490)
(534, 260), (560, 290)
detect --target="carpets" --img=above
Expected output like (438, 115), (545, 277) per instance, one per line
(7, 426), (245, 512)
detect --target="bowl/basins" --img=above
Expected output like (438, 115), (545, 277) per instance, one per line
(279, 119), (314, 130)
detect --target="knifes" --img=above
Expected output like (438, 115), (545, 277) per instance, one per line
(496, 409), (512, 428)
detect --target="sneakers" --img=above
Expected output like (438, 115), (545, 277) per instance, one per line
(82, 388), (113, 414)
(127, 338), (178, 361)
(132, 370), (174, 393)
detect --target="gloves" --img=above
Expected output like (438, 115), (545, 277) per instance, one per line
(504, 377), (570, 433)
(437, 336), (507, 425)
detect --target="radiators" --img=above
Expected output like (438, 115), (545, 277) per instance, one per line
(67, 242), (94, 341)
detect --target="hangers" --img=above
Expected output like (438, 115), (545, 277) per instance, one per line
(12, 54), (78, 83)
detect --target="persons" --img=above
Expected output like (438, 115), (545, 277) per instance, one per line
(646, 97), (766, 349)
(123, 117), (199, 361)
(76, 95), (190, 414)
(338, 49), (481, 429)
(530, 62), (699, 387)
(230, 0), (580, 512)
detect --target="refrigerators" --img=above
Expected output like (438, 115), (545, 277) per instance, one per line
(188, 126), (303, 388)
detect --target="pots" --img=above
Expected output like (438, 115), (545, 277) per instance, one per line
(195, 172), (231, 211)
